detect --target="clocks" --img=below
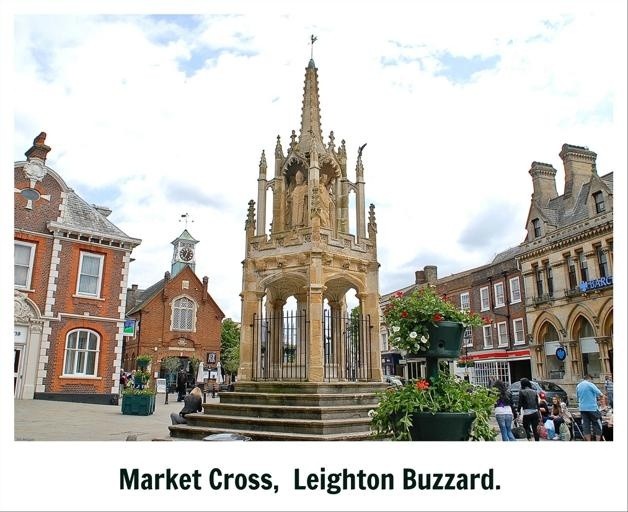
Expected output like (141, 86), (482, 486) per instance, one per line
(177, 247), (195, 264)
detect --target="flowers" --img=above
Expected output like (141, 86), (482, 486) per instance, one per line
(366, 374), (500, 441)
(382, 287), (494, 355)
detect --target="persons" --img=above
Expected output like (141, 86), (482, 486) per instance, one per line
(292, 170), (309, 232)
(318, 173), (336, 229)
(177, 370), (187, 401)
(480, 374), (613, 441)
(170, 386), (203, 426)
(120, 372), (136, 391)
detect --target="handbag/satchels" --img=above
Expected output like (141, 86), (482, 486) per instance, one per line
(559, 423), (572, 441)
(511, 427), (528, 439)
(535, 419), (555, 439)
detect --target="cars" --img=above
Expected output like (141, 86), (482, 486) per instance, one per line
(506, 379), (568, 409)
(381, 374), (406, 387)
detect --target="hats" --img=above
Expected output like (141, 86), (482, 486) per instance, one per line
(538, 392), (546, 400)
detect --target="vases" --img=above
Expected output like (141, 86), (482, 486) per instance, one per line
(411, 411), (475, 441)
(411, 320), (465, 358)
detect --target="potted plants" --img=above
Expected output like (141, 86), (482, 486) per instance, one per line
(121, 355), (156, 416)
